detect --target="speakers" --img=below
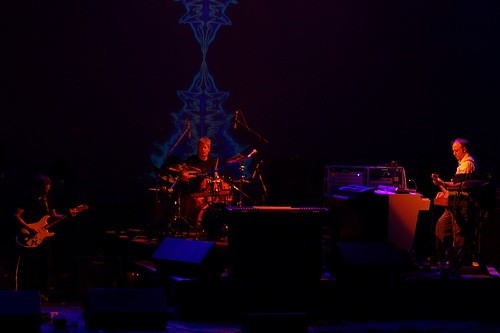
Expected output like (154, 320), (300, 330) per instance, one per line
(0, 291), (41, 333)
(83, 287), (168, 333)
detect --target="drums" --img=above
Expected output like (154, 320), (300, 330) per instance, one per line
(197, 177), (231, 196)
(196, 202), (229, 237)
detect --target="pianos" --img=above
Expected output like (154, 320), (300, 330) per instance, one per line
(432, 190), (500, 261)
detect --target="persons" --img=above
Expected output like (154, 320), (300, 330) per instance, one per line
(432, 138), (480, 275)
(184, 137), (218, 194)
(13, 175), (77, 301)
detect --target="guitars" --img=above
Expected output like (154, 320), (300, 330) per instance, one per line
(17, 203), (90, 248)
(431, 172), (452, 191)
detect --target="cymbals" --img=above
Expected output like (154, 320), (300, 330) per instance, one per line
(168, 165), (201, 173)
(151, 167), (176, 184)
(232, 180), (255, 183)
(226, 154), (253, 164)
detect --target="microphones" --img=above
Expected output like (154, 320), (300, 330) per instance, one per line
(234, 110), (239, 129)
(186, 119), (191, 139)
(248, 149), (257, 158)
